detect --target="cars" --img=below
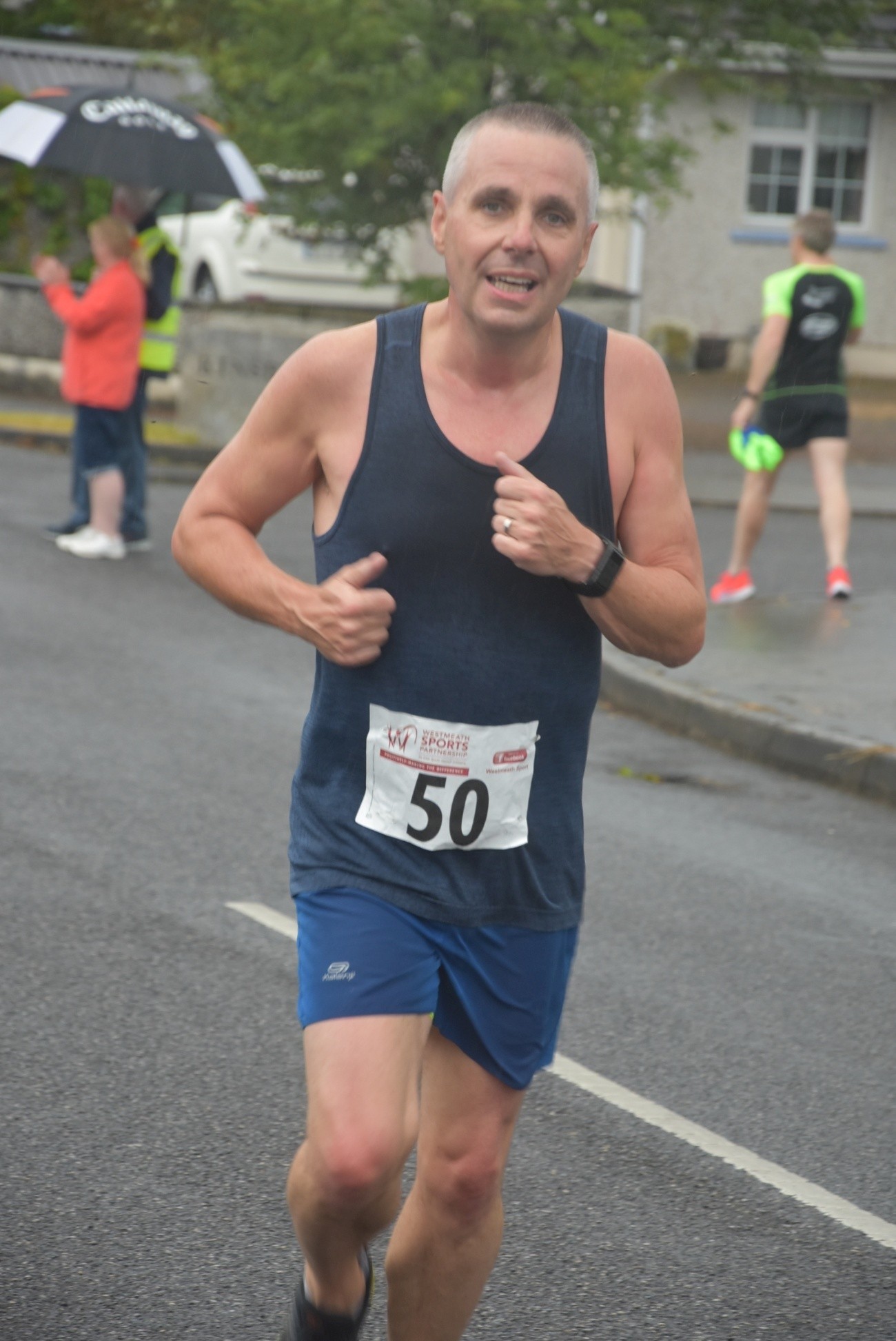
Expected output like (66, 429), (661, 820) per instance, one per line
(153, 158), (408, 316)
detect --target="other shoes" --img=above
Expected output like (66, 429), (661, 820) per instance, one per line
(279, 1242), (375, 1340)
(56, 527), (126, 560)
(709, 569), (755, 604)
(826, 565), (853, 599)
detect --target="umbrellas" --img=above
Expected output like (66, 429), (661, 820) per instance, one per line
(0, 65), (268, 232)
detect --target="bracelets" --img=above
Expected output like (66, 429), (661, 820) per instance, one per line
(741, 390), (760, 401)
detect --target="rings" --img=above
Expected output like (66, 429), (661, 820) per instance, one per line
(504, 518), (512, 533)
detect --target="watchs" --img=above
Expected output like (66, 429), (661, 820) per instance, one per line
(561, 531), (626, 598)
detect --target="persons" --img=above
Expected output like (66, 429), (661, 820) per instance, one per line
(168, 100), (707, 1341)
(41, 183), (182, 550)
(33, 211), (147, 559)
(707, 210), (868, 604)
